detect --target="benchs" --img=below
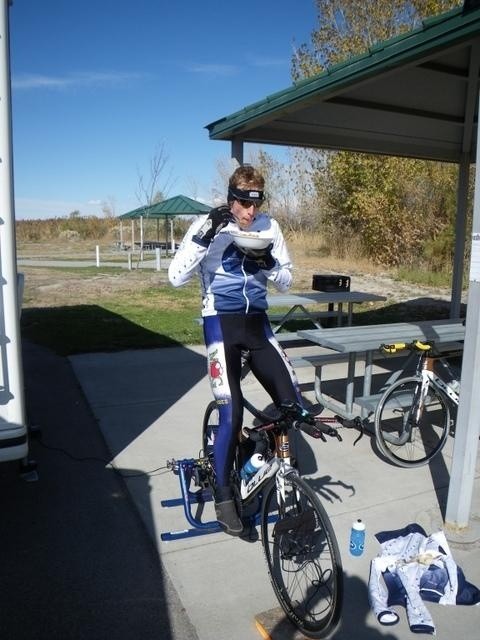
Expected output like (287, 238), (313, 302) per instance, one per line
(301, 340), (463, 368)
(274, 331), (309, 346)
(198, 310), (346, 325)
(355, 381), (459, 414)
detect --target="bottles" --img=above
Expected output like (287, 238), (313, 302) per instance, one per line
(240, 452), (264, 482)
(350, 516), (367, 556)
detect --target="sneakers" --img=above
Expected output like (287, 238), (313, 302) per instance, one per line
(213, 494), (243, 535)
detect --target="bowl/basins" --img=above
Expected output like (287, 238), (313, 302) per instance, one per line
(228, 231), (275, 250)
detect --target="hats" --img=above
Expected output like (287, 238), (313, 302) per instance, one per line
(228, 189), (265, 201)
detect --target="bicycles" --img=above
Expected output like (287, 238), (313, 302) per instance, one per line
(373, 339), (461, 467)
(202, 395), (342, 640)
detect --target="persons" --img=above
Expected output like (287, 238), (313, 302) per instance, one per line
(165, 163), (308, 536)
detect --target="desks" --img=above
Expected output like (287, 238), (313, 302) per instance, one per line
(266, 288), (387, 325)
(297, 315), (466, 446)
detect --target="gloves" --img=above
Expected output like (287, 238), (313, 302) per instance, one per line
(197, 206), (236, 243)
(239, 243), (275, 269)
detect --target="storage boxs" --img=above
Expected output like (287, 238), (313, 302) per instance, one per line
(312, 274), (350, 292)
